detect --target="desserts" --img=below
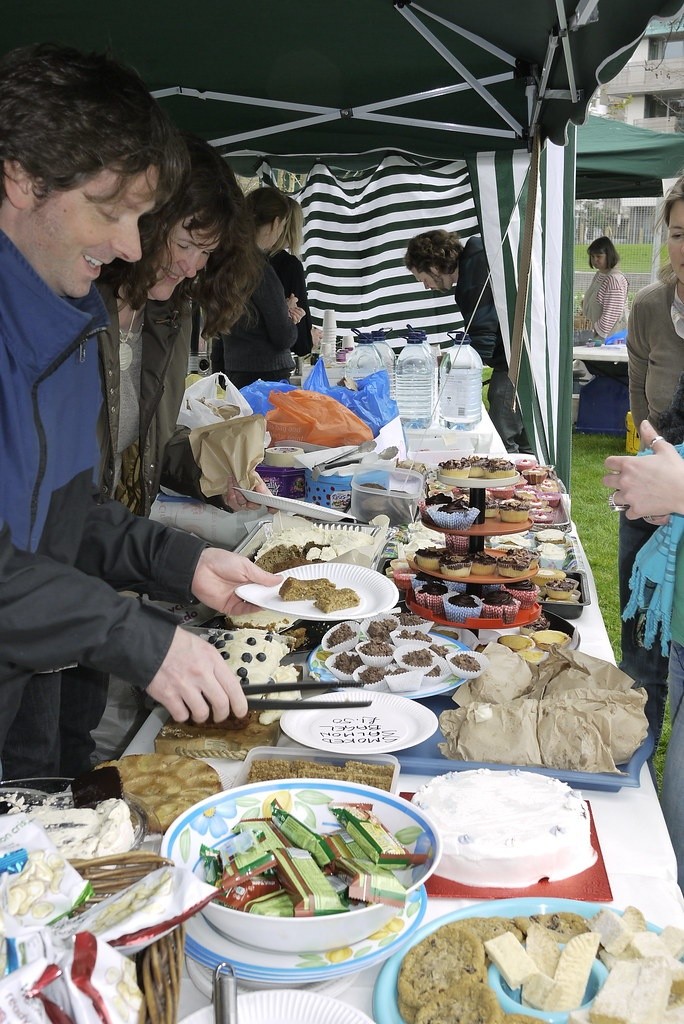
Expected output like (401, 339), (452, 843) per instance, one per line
(387, 455), (583, 665)
(321, 613), (490, 693)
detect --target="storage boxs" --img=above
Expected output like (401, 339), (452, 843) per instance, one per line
(350, 465), (425, 525)
(230, 747), (400, 791)
(405, 437), (475, 465)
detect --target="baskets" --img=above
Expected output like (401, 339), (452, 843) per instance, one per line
(304, 470), (389, 507)
(53, 852), (186, 1024)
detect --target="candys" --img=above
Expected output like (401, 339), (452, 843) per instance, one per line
(199, 799), (411, 917)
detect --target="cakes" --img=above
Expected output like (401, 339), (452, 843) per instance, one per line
(408, 768), (597, 888)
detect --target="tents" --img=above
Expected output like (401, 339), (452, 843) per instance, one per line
(0, 0), (684, 483)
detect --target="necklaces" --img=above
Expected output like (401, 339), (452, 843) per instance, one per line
(119, 310), (136, 371)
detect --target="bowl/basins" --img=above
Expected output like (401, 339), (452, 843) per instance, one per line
(350, 467), (424, 527)
(230, 746), (401, 794)
(160, 779), (442, 953)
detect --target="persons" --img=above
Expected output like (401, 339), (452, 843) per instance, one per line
(0, 59), (284, 781)
(602, 421), (684, 895)
(404, 229), (533, 455)
(580, 236), (630, 341)
(199, 187), (306, 389)
(209, 199), (313, 380)
(617, 180), (684, 792)
(98, 136), (278, 516)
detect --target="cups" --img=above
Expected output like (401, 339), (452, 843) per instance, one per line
(320, 309), (354, 366)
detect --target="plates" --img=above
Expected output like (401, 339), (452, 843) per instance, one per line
(372, 896), (684, 1024)
(399, 791), (612, 903)
(0, 774), (162, 866)
(180, 885), (428, 998)
(278, 690), (439, 756)
(308, 631), (473, 699)
(173, 991), (376, 1024)
(232, 485), (357, 524)
(234, 563), (399, 620)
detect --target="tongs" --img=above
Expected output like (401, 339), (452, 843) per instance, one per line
(312, 440), (398, 470)
(203, 681), (372, 710)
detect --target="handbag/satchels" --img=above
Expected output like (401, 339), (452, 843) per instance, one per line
(176, 372), (253, 432)
(301, 358), (399, 438)
(265, 389), (374, 447)
(237, 379), (300, 416)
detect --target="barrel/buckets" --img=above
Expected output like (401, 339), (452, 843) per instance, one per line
(625, 412), (640, 454)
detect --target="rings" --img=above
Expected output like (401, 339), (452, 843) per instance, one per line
(644, 515), (654, 523)
(650, 436), (665, 448)
(609, 491), (627, 512)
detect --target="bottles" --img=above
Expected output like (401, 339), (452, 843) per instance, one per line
(344, 323), (482, 429)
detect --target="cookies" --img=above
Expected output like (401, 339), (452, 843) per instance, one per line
(396, 906), (684, 1024)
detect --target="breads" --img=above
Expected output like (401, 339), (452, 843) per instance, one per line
(254, 541), (361, 613)
(91, 610), (394, 834)
